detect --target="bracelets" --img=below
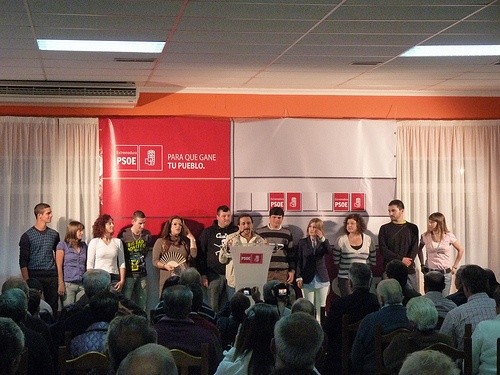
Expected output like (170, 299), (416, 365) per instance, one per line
(421, 264), (424, 266)
(163, 264), (165, 269)
(320, 235), (323, 239)
(453, 266), (457, 270)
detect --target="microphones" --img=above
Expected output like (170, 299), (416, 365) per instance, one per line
(227, 230), (245, 254)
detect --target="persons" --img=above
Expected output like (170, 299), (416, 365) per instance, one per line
(55, 221), (88, 312)
(19, 203), (61, 314)
(378, 200), (419, 291)
(256, 207), (295, 286)
(152, 216), (197, 299)
(0, 260), (500, 375)
(417, 212), (464, 298)
(196, 205), (240, 312)
(295, 218), (332, 324)
(332, 214), (377, 299)
(86, 214), (126, 292)
(219, 214), (264, 301)
(117, 211), (153, 313)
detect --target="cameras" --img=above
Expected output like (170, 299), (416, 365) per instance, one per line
(242, 287), (256, 296)
(271, 282), (290, 298)
